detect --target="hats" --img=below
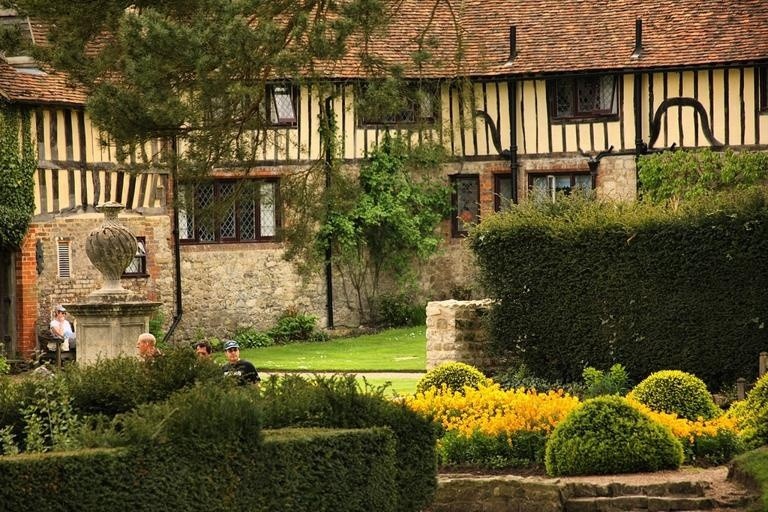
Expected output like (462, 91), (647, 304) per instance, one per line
(57, 307), (66, 312)
(224, 341), (239, 351)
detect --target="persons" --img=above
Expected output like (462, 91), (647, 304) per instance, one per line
(194, 342), (212, 359)
(136, 332), (161, 365)
(50, 306), (76, 351)
(222, 340), (262, 388)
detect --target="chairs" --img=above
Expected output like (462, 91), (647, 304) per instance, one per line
(33, 316), (76, 368)
